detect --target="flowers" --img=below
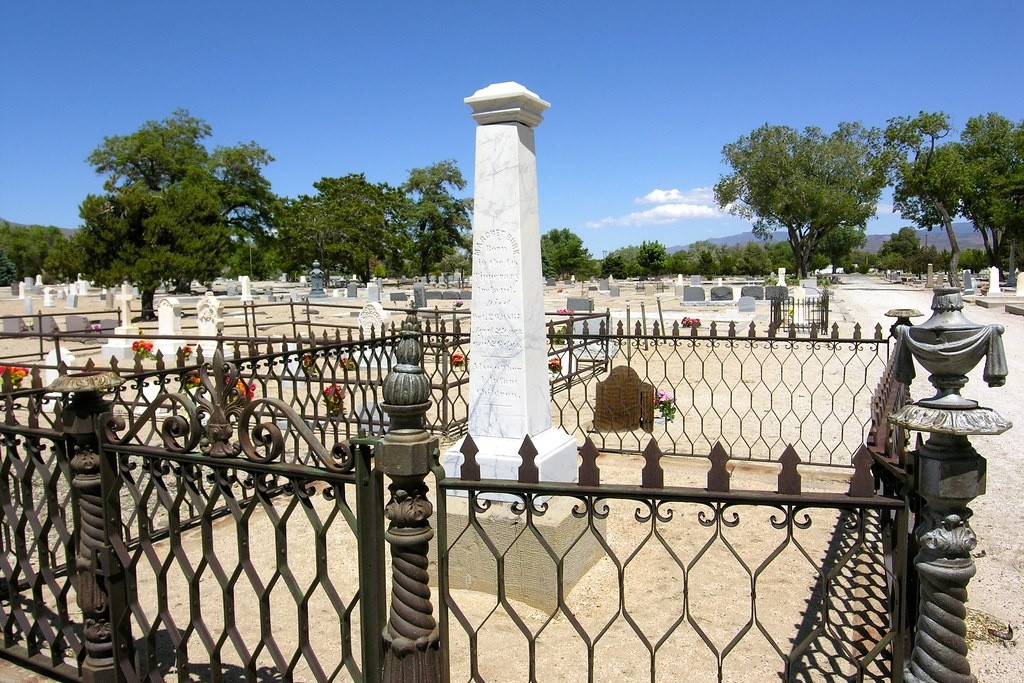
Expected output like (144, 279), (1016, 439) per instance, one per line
(681, 317), (701, 328)
(322, 385), (346, 416)
(451, 354), (469, 367)
(454, 301), (463, 308)
(553, 309), (574, 345)
(299, 353), (318, 379)
(338, 358), (357, 371)
(179, 345), (206, 388)
(549, 357), (563, 374)
(653, 388), (677, 422)
(223, 376), (256, 404)
(0, 365), (30, 392)
(130, 340), (158, 361)
